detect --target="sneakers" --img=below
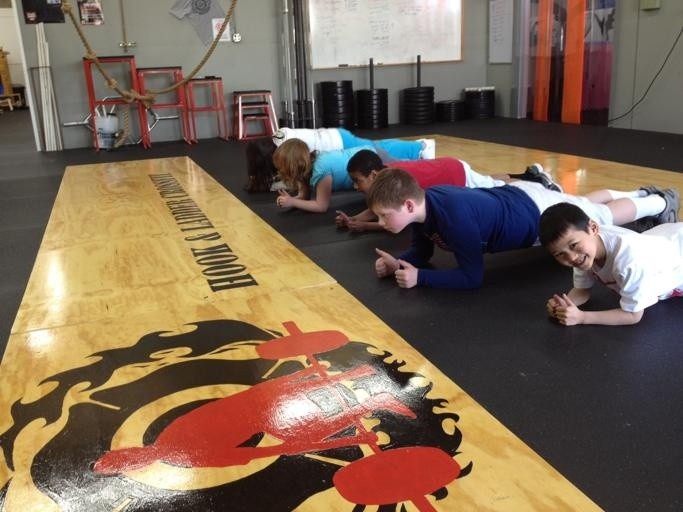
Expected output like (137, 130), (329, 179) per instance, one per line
(414, 138), (436, 160)
(523, 162), (544, 178)
(532, 171), (563, 194)
(639, 184), (663, 195)
(652, 187), (681, 230)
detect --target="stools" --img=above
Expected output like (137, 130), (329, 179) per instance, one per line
(80, 52), (281, 154)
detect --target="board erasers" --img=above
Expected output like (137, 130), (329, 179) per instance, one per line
(338, 64), (348, 66)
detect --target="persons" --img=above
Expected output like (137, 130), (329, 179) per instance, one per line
(244, 127), (435, 194)
(537, 201), (683, 327)
(366, 166), (679, 290)
(335, 148), (563, 234)
(271, 138), (399, 213)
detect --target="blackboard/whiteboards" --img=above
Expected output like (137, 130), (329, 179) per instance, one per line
(304, 0), (464, 70)
(489, 1), (514, 65)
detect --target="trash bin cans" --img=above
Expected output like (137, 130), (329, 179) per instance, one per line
(94, 116), (120, 149)
(12, 85), (25, 110)
